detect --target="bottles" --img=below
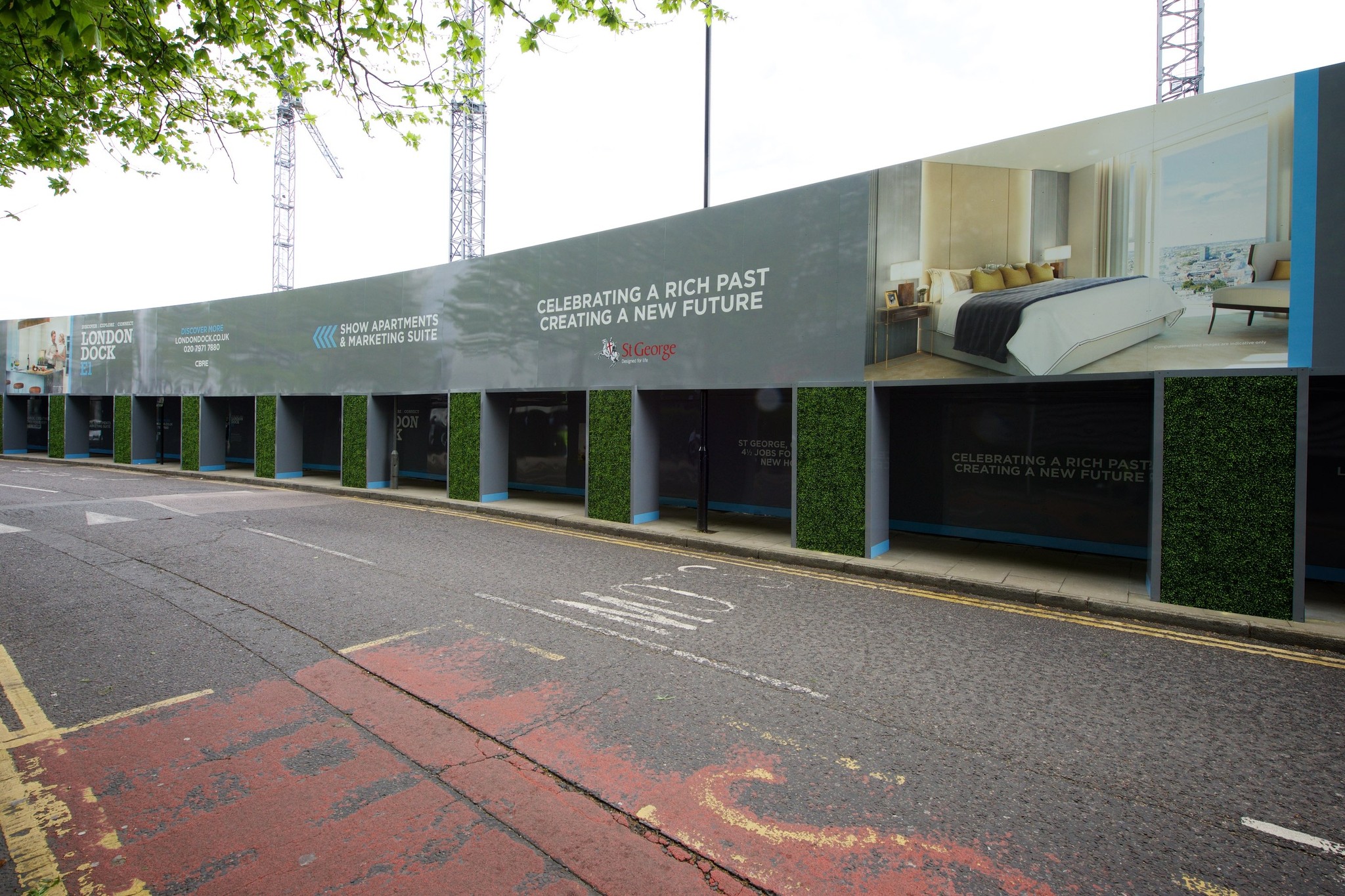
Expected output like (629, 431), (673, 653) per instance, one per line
(27, 353), (29, 370)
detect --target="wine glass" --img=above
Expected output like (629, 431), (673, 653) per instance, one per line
(14, 360), (20, 368)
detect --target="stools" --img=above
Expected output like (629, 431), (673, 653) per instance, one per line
(6, 380), (41, 394)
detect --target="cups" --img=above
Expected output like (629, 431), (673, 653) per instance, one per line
(10, 357), (14, 366)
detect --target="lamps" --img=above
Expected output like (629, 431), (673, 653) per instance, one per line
(1046, 244), (1072, 278)
(889, 259), (924, 305)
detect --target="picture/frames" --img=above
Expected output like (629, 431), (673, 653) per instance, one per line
(883, 290), (899, 309)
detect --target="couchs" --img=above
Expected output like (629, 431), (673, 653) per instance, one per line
(1205, 240), (1291, 334)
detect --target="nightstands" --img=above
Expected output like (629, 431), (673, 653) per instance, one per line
(877, 304), (934, 368)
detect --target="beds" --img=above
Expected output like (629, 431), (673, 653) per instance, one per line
(922, 261), (1187, 376)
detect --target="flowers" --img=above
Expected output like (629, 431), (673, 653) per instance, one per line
(916, 283), (929, 296)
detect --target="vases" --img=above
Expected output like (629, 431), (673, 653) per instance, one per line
(918, 294), (925, 302)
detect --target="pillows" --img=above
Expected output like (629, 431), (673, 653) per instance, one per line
(925, 268), (946, 304)
(999, 266), (1031, 289)
(970, 267), (1006, 293)
(1025, 263), (1054, 285)
(938, 268), (983, 304)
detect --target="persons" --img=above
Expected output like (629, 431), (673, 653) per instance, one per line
(54, 334), (67, 393)
(890, 296), (894, 304)
(44, 330), (58, 368)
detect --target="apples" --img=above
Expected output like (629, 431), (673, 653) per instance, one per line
(33, 365), (46, 371)
